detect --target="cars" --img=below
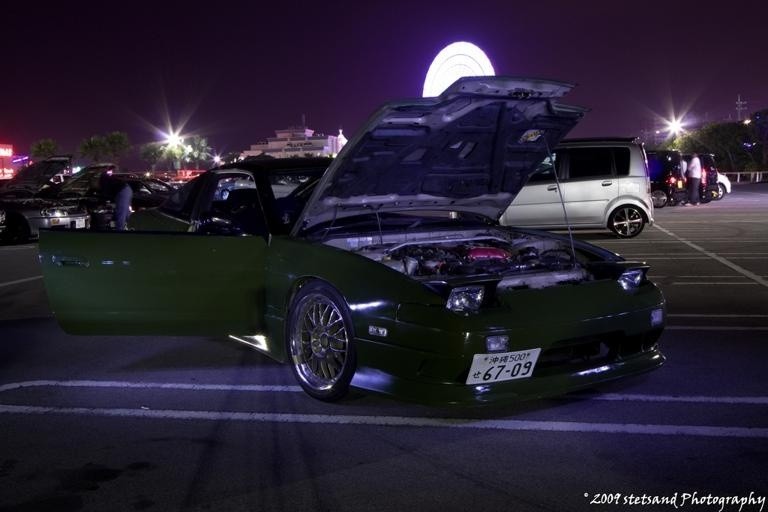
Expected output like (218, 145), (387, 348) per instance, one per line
(715, 171), (731, 200)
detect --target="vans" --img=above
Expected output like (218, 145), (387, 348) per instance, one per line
(645, 148), (719, 208)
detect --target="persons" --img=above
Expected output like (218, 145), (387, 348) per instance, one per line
(684, 151), (701, 207)
